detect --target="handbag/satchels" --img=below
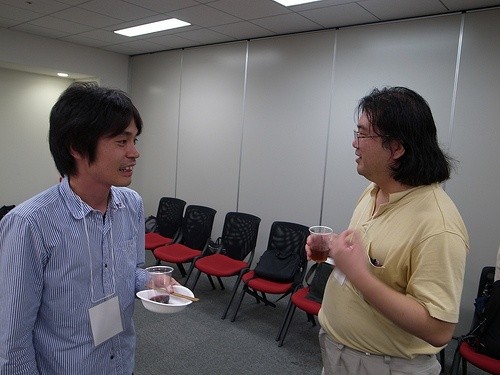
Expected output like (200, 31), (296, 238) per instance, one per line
(255, 249), (301, 283)
(466, 280), (500, 361)
(304, 262), (334, 303)
(203, 237), (226, 256)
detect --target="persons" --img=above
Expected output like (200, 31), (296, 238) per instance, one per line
(305, 85), (470, 375)
(0, 80), (182, 375)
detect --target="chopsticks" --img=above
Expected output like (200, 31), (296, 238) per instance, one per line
(144, 284), (199, 301)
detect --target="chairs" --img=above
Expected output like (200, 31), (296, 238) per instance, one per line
(230, 220), (311, 342)
(190, 212), (261, 321)
(277, 255), (334, 348)
(449, 266), (500, 375)
(144, 195), (187, 277)
(153, 204), (217, 291)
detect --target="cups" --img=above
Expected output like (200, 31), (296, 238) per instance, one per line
(309, 226), (333, 262)
(145, 265), (174, 304)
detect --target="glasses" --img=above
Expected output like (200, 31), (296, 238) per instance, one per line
(354, 130), (390, 147)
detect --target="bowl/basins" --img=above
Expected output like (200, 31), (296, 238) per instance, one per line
(135, 285), (194, 314)
